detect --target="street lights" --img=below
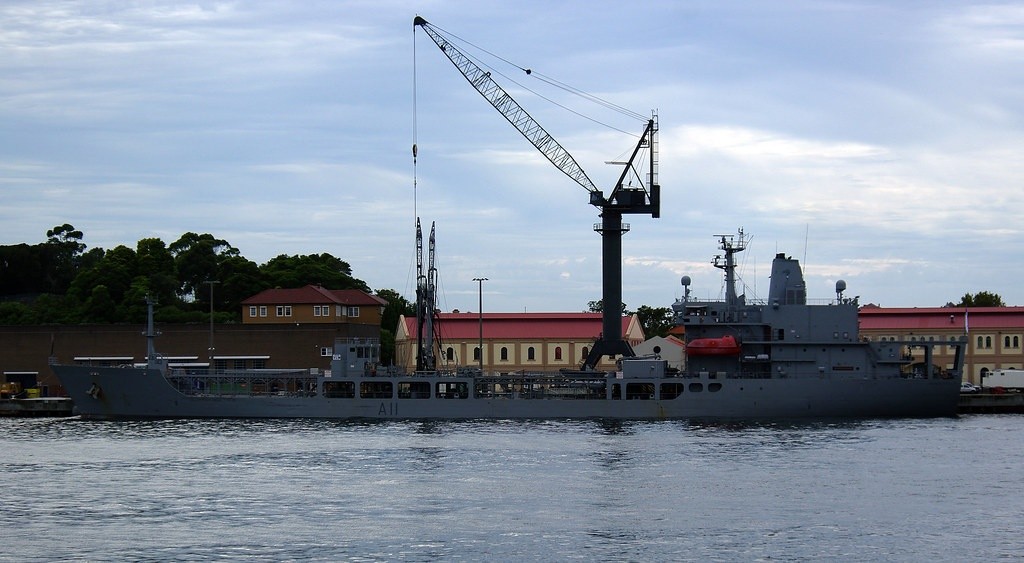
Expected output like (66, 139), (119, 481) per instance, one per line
(204, 281), (221, 395)
(473, 278), (488, 369)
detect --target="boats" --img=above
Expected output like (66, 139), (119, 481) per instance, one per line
(559, 368), (607, 379)
(0, 372), (73, 418)
(48, 235), (970, 421)
(687, 335), (741, 356)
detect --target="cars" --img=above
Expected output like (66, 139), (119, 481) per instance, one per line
(962, 382), (982, 391)
(960, 384), (976, 392)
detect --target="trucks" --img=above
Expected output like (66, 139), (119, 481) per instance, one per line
(983, 370), (1024, 393)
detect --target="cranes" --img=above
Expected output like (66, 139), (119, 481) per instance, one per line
(412, 16), (660, 371)
(415, 216), (441, 375)
